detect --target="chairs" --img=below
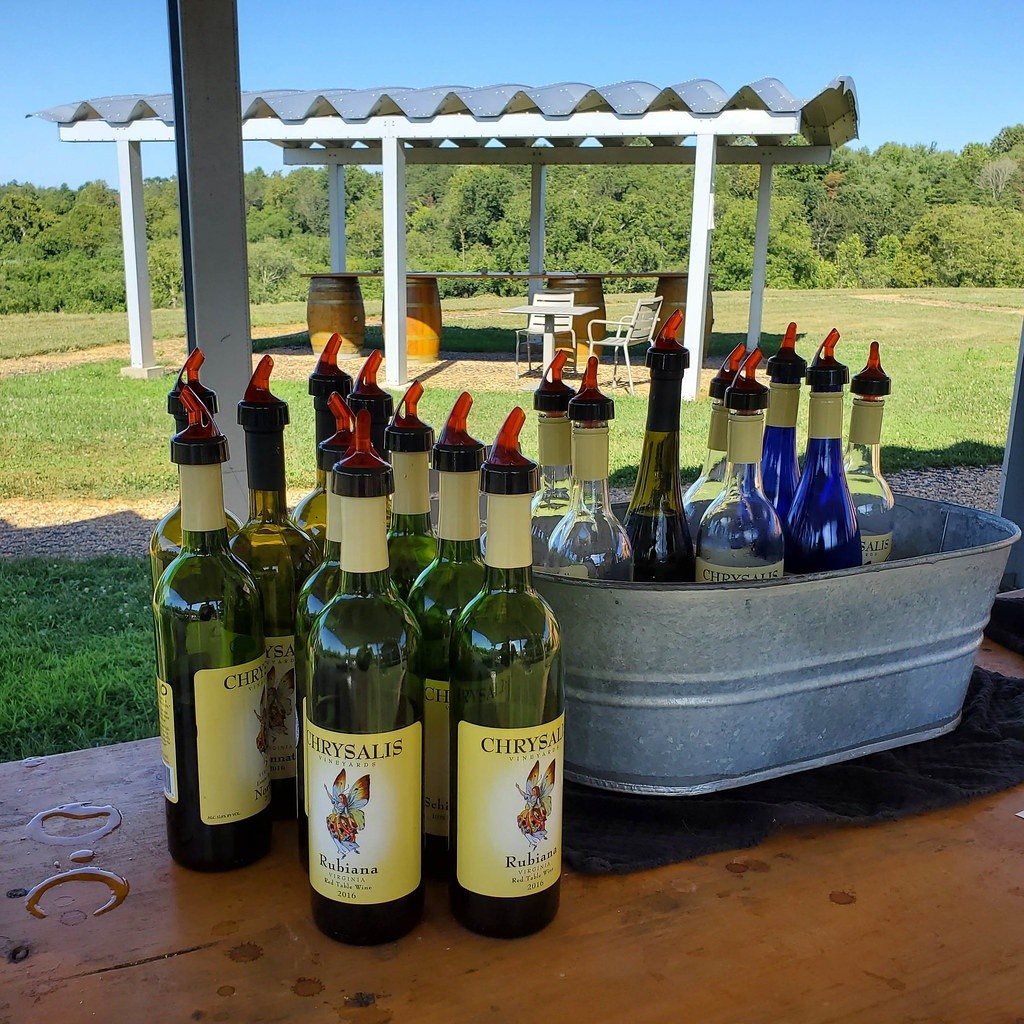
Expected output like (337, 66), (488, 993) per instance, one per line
(585, 295), (663, 395)
(515, 290), (577, 378)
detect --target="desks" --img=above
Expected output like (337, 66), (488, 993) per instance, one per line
(500, 305), (600, 391)
(1, 591), (1024, 1022)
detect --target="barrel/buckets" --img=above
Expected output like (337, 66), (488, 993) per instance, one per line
(381, 278), (441, 361)
(307, 277), (365, 354)
(547, 277), (606, 371)
(654, 277), (714, 352)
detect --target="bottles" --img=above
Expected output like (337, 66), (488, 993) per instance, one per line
(782, 328), (863, 579)
(543, 355), (633, 581)
(681, 343), (747, 544)
(694, 348), (785, 580)
(759, 321), (808, 526)
(844, 341), (895, 565)
(623, 310), (694, 584)
(150, 330), (575, 946)
